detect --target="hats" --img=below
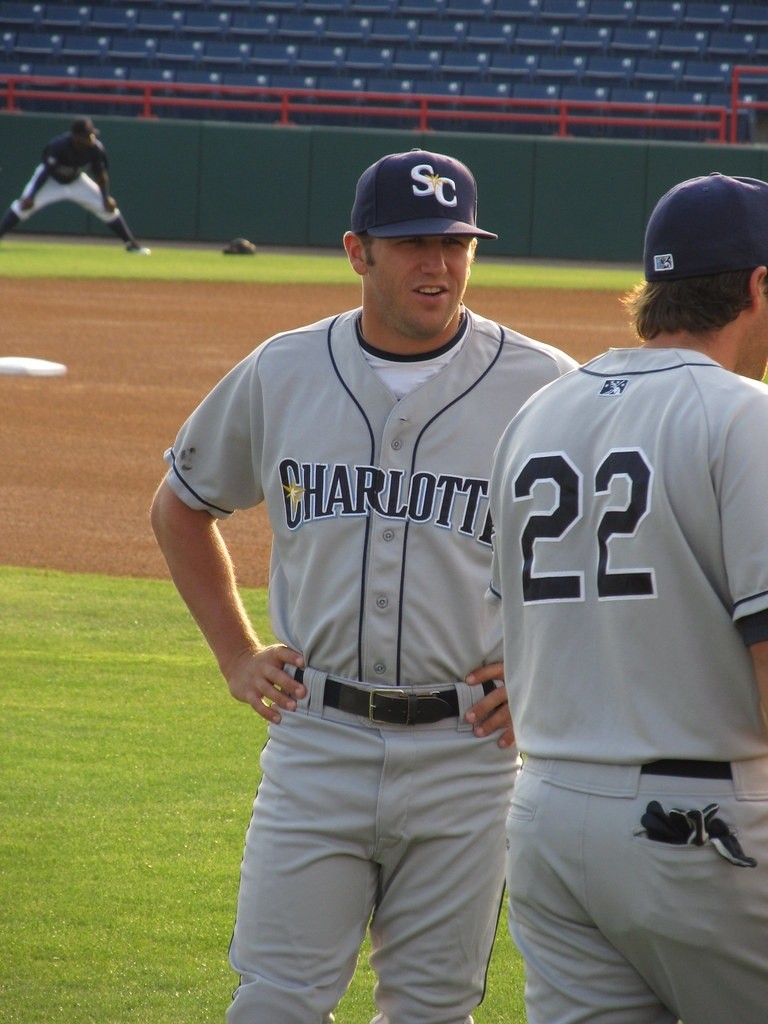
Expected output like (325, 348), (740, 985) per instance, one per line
(643, 172), (768, 282)
(351, 148), (498, 239)
(72, 118), (100, 137)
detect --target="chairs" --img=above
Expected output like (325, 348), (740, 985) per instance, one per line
(0, 0), (768, 96)
(511, 83), (559, 136)
(365, 78), (413, 130)
(607, 89), (655, 140)
(413, 79), (460, 131)
(561, 85), (606, 138)
(655, 91), (706, 142)
(705, 93), (757, 143)
(461, 82), (508, 133)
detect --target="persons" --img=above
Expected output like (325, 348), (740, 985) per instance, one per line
(0, 114), (152, 255)
(484, 171), (767, 1024)
(148, 149), (581, 1023)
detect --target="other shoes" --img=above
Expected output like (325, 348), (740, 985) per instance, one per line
(128, 246), (151, 255)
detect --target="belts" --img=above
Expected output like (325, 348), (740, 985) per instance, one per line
(641, 759), (734, 780)
(295, 669), (498, 724)
